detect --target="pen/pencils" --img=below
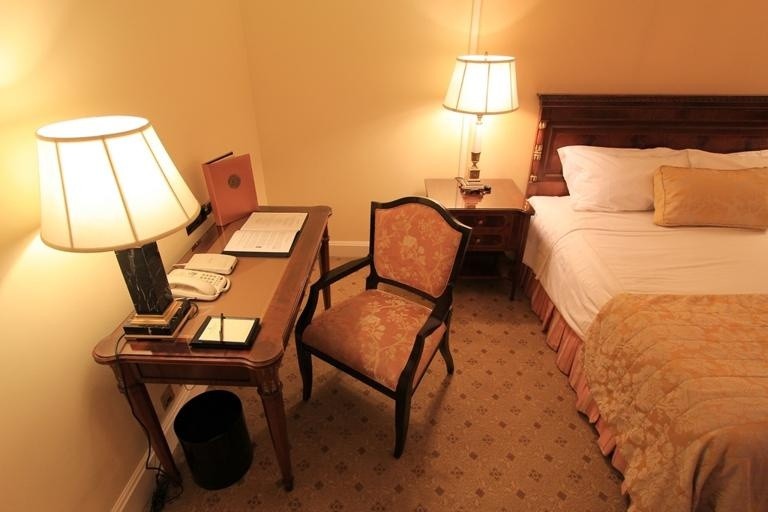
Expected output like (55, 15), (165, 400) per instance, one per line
(220, 313), (223, 343)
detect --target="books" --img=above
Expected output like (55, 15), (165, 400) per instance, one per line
(190, 315), (259, 349)
(223, 212), (308, 258)
(202, 152), (258, 226)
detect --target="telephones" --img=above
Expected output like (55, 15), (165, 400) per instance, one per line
(166, 269), (230, 301)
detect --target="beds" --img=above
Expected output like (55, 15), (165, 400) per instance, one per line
(522, 94), (767, 511)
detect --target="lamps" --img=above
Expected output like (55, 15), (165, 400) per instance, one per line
(34, 114), (202, 345)
(442, 53), (519, 194)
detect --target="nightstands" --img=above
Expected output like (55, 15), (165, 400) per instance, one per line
(425, 178), (535, 304)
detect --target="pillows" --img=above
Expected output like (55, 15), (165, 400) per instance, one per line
(555, 146), (768, 231)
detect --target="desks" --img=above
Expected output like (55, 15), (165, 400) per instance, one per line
(87, 206), (336, 497)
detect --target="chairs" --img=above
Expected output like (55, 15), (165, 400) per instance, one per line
(293, 195), (473, 466)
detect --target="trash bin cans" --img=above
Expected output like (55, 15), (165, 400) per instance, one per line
(174, 390), (253, 491)
